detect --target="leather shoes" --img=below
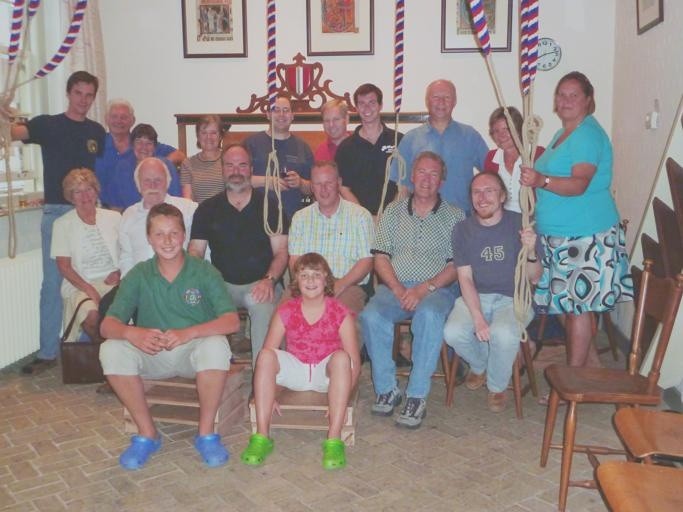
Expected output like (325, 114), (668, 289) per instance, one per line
(395, 350), (409, 368)
(444, 360), (507, 414)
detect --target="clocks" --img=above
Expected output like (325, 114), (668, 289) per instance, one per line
(537, 38), (561, 71)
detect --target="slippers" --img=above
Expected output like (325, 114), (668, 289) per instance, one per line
(191, 433), (229, 468)
(119, 433), (161, 471)
(538, 393), (566, 406)
(240, 433), (273, 465)
(322, 439), (344, 470)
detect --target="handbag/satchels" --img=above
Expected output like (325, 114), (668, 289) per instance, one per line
(59, 297), (104, 385)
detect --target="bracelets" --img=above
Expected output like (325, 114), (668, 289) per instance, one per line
(526, 254), (539, 263)
(263, 276), (277, 285)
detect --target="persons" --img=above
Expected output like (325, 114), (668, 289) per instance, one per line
(442, 171), (546, 412)
(99, 203), (239, 470)
(483, 106), (547, 214)
(240, 251), (360, 470)
(50, 167), (122, 392)
(1, 70), (104, 374)
(116, 157), (215, 277)
(390, 79), (490, 216)
(317, 83), (404, 216)
(519, 72), (635, 406)
(186, 143), (282, 392)
(202, 7), (226, 32)
(94, 92), (317, 224)
(360, 149), (466, 429)
(284, 162), (375, 370)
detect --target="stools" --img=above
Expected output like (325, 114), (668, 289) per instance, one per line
(225, 308), (251, 354)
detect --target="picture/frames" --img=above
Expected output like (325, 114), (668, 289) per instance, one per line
(181, 0), (248, 58)
(636, 0), (664, 35)
(306, 0), (374, 56)
(441, 0), (513, 53)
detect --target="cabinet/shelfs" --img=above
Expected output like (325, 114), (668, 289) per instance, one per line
(630, 113), (683, 358)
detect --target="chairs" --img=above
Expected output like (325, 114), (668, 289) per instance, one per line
(538, 256), (683, 511)
(388, 314), (454, 409)
(536, 256), (621, 361)
(445, 314), (537, 420)
(597, 459), (683, 512)
(611, 406), (683, 466)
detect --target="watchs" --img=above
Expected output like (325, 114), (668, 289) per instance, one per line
(541, 177), (549, 190)
(425, 281), (435, 294)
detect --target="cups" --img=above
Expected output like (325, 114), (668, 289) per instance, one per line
(279, 167), (289, 178)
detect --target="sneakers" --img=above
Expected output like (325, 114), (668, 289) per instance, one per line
(232, 338), (251, 354)
(370, 388), (401, 417)
(95, 383), (114, 395)
(23, 358), (57, 375)
(394, 398), (426, 429)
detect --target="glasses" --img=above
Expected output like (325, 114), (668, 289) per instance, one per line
(274, 106), (289, 113)
